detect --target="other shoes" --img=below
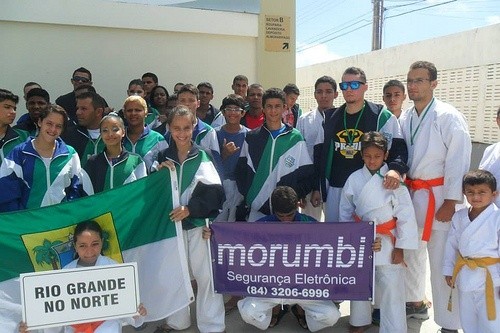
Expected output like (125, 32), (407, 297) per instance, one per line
(130, 296), (243, 333)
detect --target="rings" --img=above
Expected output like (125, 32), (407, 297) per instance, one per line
(394, 183), (397, 185)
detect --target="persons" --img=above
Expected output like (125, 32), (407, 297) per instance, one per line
(79, 110), (151, 330)
(399, 60), (475, 333)
(236, 87), (317, 224)
(61, 91), (110, 163)
(0, 88), (36, 168)
(295, 73), (340, 222)
(311, 66), (411, 243)
(118, 94), (169, 178)
(163, 82), (224, 177)
(147, 102), (228, 333)
(15, 63), (303, 134)
(475, 107), (500, 211)
(441, 169), (500, 333)
(0, 104), (82, 214)
(200, 184), (384, 333)
(334, 130), (420, 333)
(213, 93), (253, 315)
(15, 219), (147, 333)
(380, 78), (409, 127)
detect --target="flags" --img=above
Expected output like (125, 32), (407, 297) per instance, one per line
(0, 162), (195, 332)
(206, 217), (378, 304)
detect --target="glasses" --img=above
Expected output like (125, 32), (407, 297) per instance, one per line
(339, 81), (365, 90)
(73, 76), (90, 83)
(406, 77), (432, 84)
(224, 107), (243, 113)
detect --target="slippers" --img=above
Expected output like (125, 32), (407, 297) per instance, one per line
(292, 304), (308, 329)
(269, 304), (289, 327)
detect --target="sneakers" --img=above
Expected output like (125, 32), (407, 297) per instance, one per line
(406, 302), (429, 320)
